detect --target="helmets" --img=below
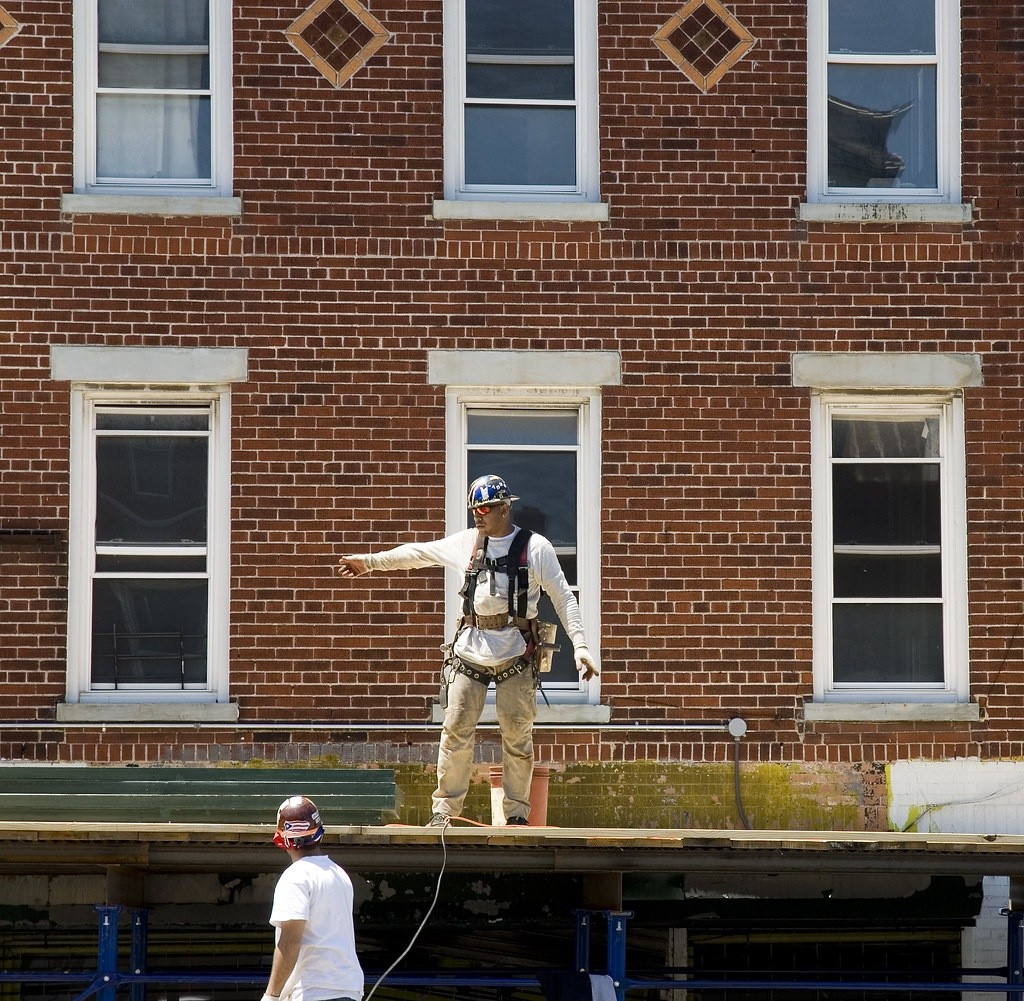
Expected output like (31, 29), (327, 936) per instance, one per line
(273, 797), (324, 850)
(467, 475), (520, 508)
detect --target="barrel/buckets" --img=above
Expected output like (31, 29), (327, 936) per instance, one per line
(489, 765), (550, 827)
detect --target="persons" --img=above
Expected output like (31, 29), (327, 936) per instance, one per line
(337, 474), (599, 827)
(260, 796), (366, 1000)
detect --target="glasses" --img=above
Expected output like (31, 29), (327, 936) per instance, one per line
(472, 504), (504, 516)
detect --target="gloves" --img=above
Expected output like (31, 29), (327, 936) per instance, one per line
(573, 646), (600, 681)
(337, 554), (368, 578)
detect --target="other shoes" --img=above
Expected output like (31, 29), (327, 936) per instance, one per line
(427, 812), (452, 829)
(506, 816), (528, 826)
